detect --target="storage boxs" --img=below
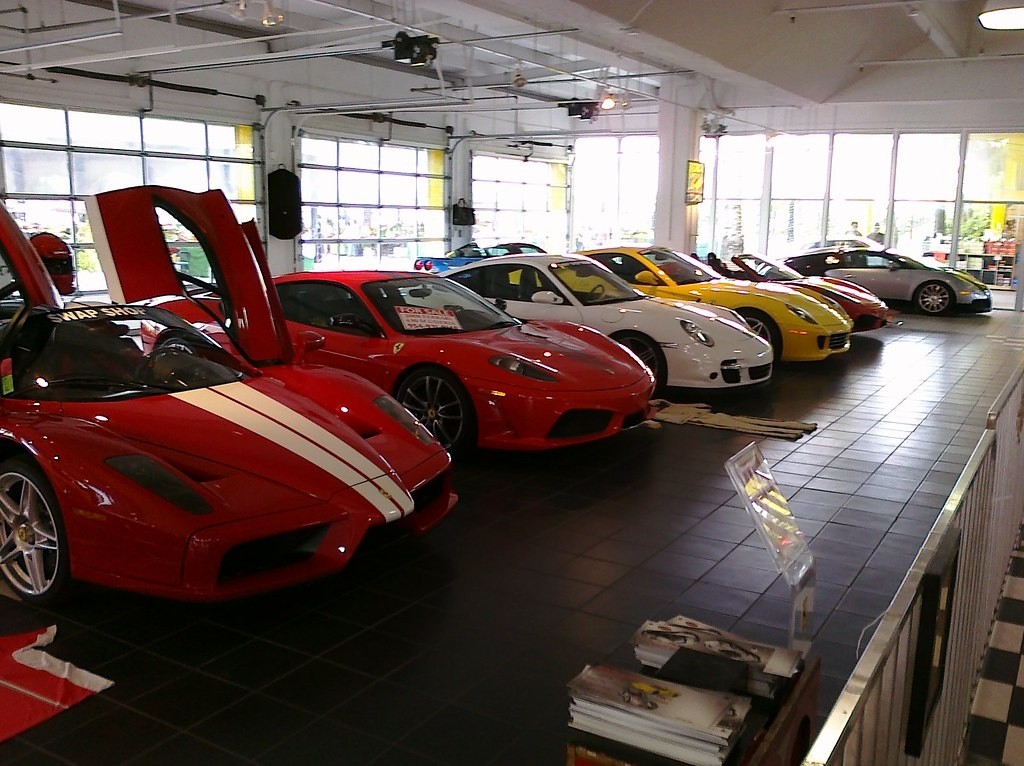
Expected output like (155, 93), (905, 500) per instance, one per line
(934, 241), (1015, 269)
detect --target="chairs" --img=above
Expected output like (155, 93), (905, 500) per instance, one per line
(354, 288), (391, 328)
(707, 252), (725, 275)
(855, 256), (867, 267)
(519, 269), (537, 302)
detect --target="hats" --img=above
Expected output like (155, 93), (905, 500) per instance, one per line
(874, 223), (880, 229)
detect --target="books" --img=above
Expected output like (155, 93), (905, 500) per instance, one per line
(568, 616), (804, 766)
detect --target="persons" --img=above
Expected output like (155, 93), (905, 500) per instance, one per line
(867, 222), (884, 245)
(845, 222), (862, 236)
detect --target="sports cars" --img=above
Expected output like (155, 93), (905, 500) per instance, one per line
(396, 252), (775, 402)
(800, 233), (948, 272)
(139, 269), (670, 465)
(657, 251), (894, 336)
(508, 244), (855, 368)
(1, 179), (463, 611)
(412, 241), (549, 279)
(772, 244), (993, 318)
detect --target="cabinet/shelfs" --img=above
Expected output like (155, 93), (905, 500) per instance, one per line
(924, 251), (1016, 291)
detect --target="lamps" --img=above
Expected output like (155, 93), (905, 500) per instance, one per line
(262, 0), (282, 27)
(231, 3), (247, 21)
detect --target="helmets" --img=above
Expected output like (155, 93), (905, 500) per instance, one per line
(30, 232), (76, 295)
